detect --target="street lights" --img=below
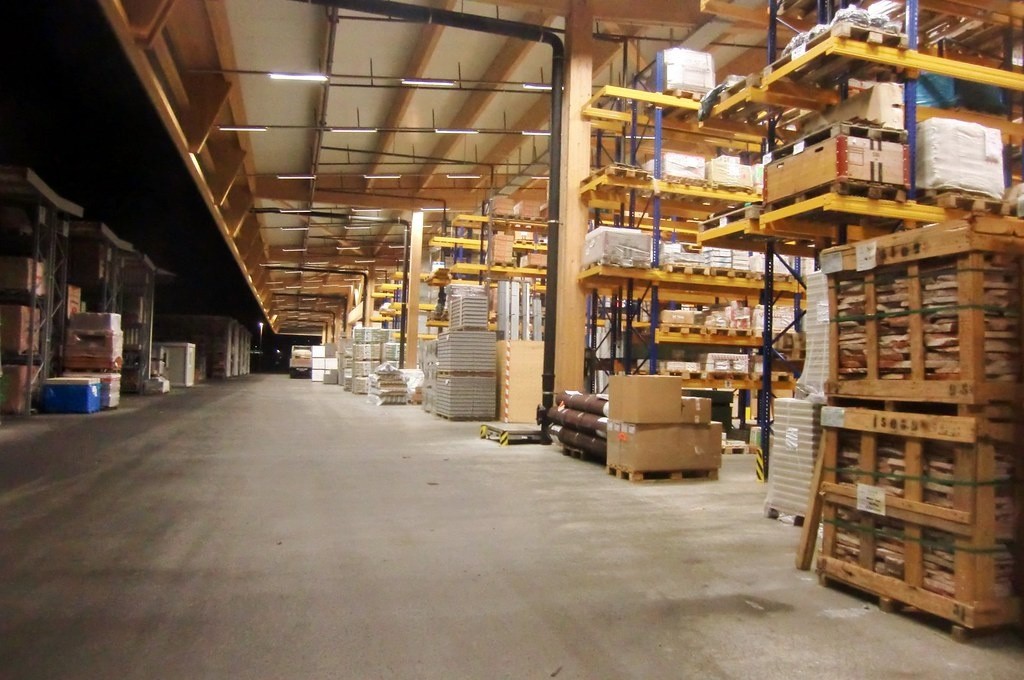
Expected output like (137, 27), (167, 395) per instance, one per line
(259, 323), (263, 352)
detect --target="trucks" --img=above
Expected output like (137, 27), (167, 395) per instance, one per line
(290, 346), (312, 379)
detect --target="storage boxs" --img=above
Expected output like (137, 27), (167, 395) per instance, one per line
(495, 340), (544, 424)
(491, 196), (540, 218)
(762, 134), (911, 204)
(492, 235), (513, 263)
(43, 382), (101, 415)
(659, 309), (694, 324)
(0, 257), (45, 414)
(66, 285), (81, 319)
(520, 253), (548, 268)
(607, 375), (723, 469)
